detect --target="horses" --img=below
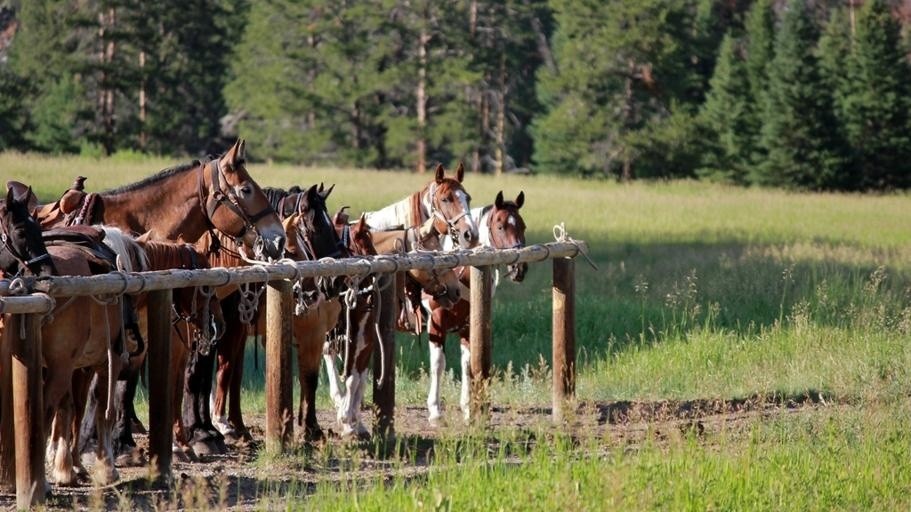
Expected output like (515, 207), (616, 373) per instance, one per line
(0, 137), (531, 482)
